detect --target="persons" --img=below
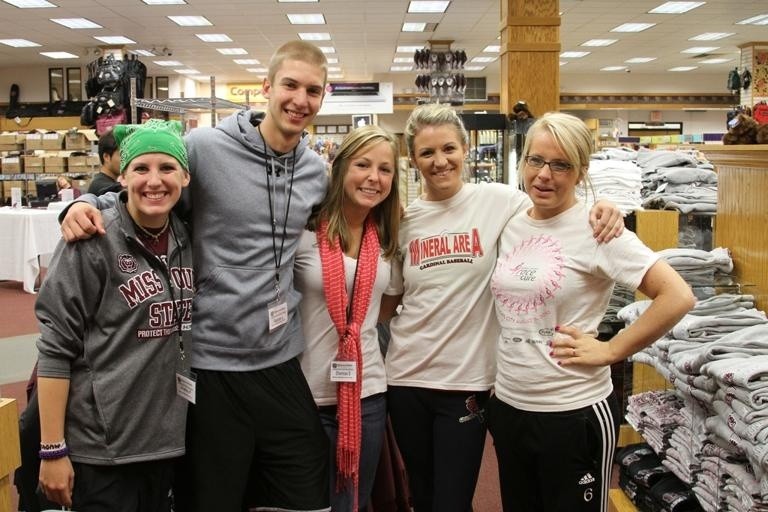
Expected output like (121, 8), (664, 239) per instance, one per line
(486, 109), (696, 512)
(85, 129), (127, 195)
(57, 38), (331, 512)
(32, 117), (195, 511)
(378, 103), (624, 512)
(287, 124), (405, 512)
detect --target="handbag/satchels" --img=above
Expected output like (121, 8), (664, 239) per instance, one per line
(727, 68), (768, 130)
(81, 53), (146, 135)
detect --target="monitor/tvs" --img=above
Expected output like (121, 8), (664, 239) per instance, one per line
(35, 181), (58, 198)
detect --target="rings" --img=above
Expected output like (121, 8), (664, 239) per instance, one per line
(572, 347), (575, 357)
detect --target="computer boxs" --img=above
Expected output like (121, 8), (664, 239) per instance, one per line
(31, 198), (60, 208)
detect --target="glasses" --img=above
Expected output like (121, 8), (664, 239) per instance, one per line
(524, 156), (573, 174)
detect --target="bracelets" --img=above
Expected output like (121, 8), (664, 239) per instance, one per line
(39, 439), (67, 451)
(37, 448), (71, 459)
